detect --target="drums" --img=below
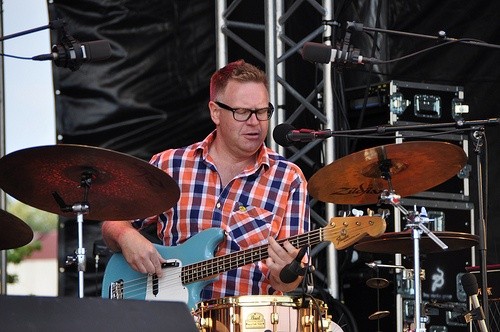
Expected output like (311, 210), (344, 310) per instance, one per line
(190, 295), (332, 332)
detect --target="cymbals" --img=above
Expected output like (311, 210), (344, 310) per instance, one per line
(0, 144), (181, 221)
(366, 278), (389, 289)
(352, 230), (480, 255)
(0, 208), (34, 251)
(307, 141), (468, 205)
(368, 311), (390, 320)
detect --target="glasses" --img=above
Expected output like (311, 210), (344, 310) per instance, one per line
(215, 102), (274, 122)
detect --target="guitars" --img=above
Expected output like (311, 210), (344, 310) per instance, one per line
(102, 207), (387, 312)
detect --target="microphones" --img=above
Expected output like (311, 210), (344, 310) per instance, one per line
(32, 39), (112, 67)
(0, 19), (64, 41)
(273, 123), (331, 147)
(279, 245), (309, 283)
(302, 42), (376, 64)
(461, 273), (488, 332)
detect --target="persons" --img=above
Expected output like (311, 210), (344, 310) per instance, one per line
(102, 59), (311, 302)
